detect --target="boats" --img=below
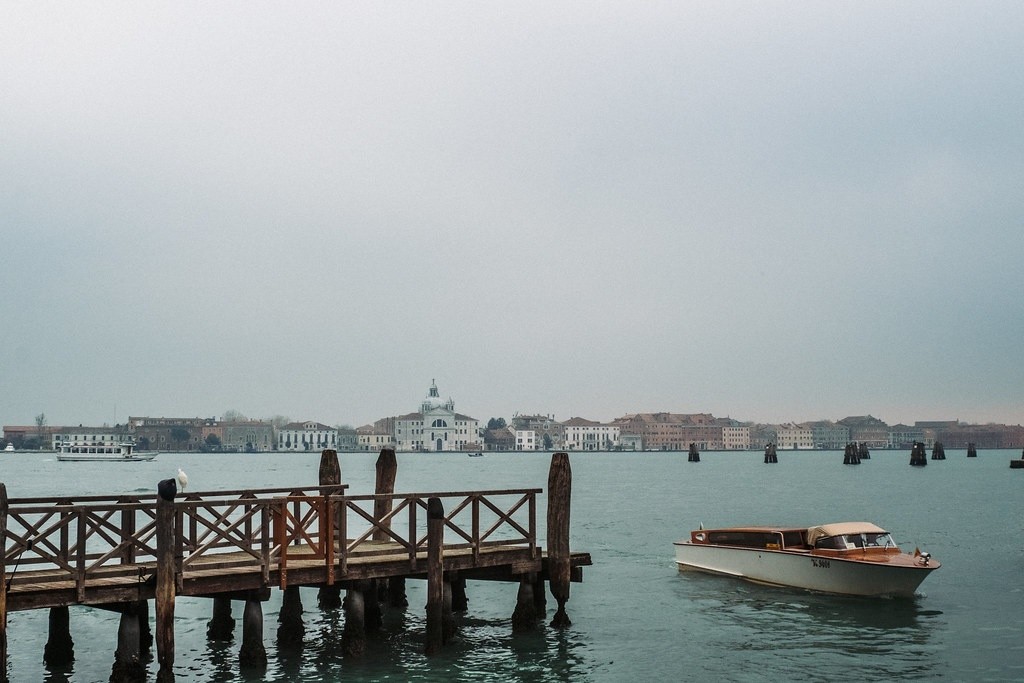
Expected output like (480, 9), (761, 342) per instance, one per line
(468, 453), (485, 457)
(5, 445), (14, 454)
(672, 521), (942, 600)
(57, 439), (159, 460)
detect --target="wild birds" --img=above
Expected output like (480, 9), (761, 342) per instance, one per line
(177, 467), (188, 493)
